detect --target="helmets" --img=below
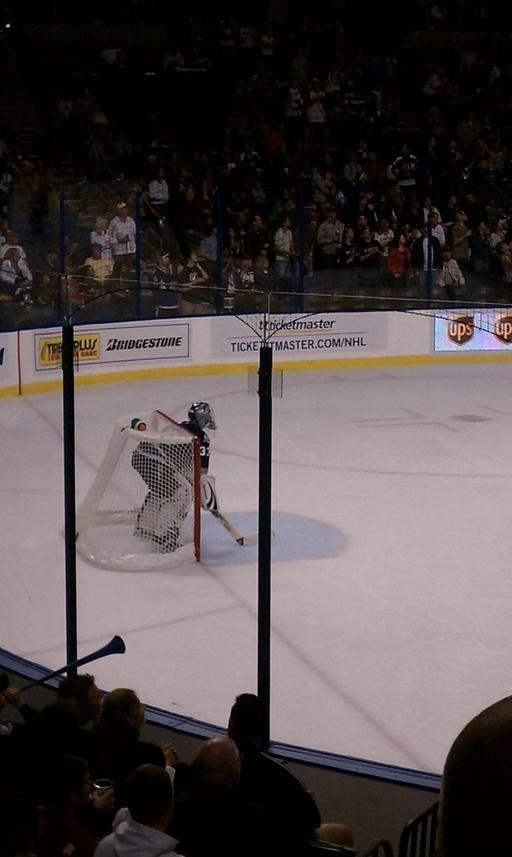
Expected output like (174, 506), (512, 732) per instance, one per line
(188, 402), (211, 415)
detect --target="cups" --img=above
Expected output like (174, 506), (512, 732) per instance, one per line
(92, 778), (113, 792)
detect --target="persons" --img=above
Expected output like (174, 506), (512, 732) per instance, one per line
(131, 401), (219, 548)
(438, 695), (512, 857)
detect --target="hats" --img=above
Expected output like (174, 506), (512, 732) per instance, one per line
(117, 202), (127, 209)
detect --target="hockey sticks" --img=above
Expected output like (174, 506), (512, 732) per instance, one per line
(156, 443), (276, 545)
